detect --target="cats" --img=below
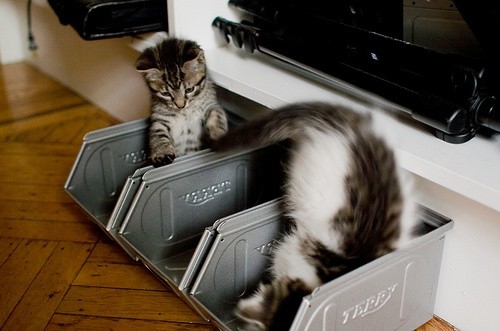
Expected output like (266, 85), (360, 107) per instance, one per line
(134, 35), (227, 168)
(209, 101), (428, 331)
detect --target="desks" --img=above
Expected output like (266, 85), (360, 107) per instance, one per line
(0, 61), (457, 331)
